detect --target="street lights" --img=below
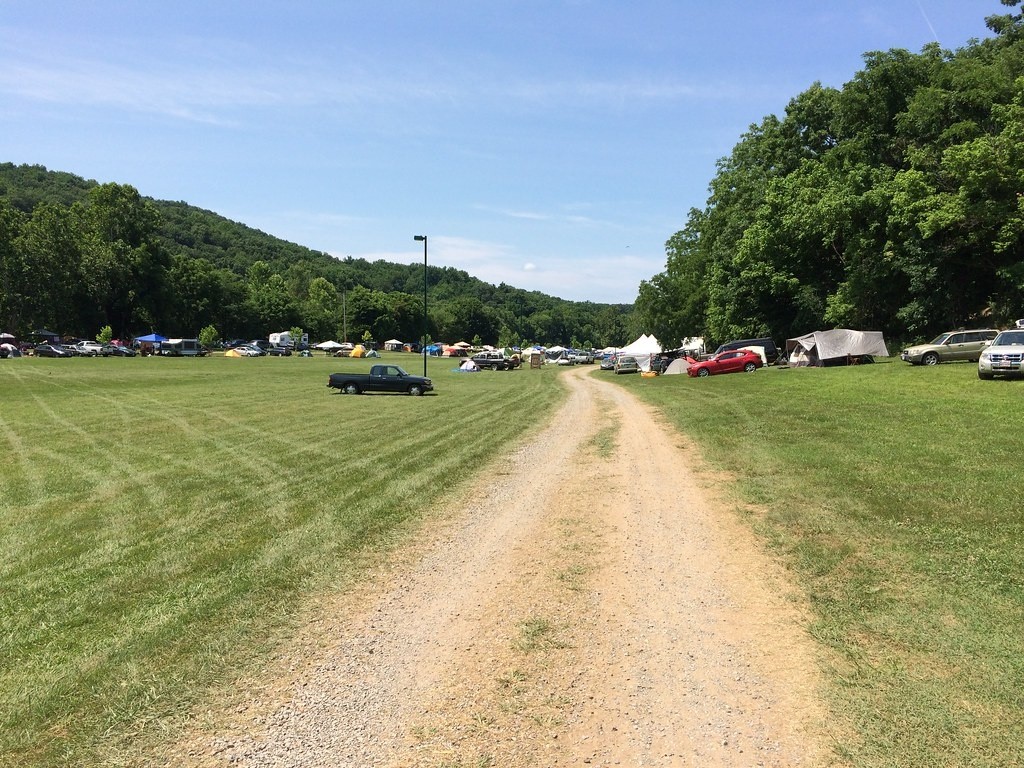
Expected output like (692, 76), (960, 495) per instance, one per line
(413, 235), (428, 377)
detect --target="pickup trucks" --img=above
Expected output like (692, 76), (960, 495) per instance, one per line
(326, 364), (434, 396)
(474, 350), (516, 369)
(568, 351), (595, 365)
(458, 353), (505, 371)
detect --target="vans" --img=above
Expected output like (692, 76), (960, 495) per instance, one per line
(710, 337), (779, 364)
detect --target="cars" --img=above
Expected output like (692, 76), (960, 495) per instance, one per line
(32, 344), (72, 357)
(900, 327), (1003, 366)
(978, 327), (1024, 380)
(61, 340), (136, 357)
(250, 346), (268, 356)
(270, 347), (291, 356)
(557, 357), (574, 366)
(600, 356), (638, 374)
(232, 346), (258, 357)
(685, 350), (764, 377)
(249, 340), (265, 350)
